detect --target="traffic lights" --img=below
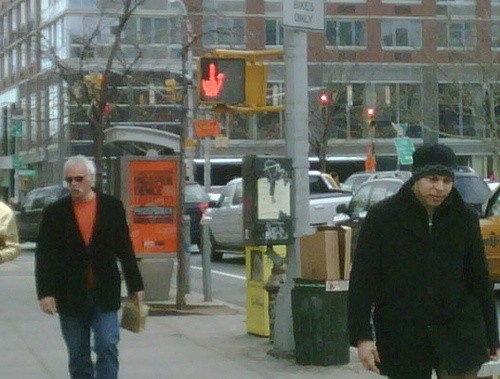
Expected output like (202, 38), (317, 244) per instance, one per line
(196, 55), (246, 105)
(366, 107), (377, 127)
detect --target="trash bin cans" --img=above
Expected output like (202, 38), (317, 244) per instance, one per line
(290, 278), (350, 366)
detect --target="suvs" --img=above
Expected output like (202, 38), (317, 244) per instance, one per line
(336, 165), (492, 262)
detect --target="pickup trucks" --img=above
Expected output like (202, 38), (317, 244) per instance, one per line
(199, 170), (354, 261)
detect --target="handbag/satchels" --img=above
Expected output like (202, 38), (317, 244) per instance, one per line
(120, 298), (149, 333)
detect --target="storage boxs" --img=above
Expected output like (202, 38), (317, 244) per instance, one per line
(299, 225), (340, 282)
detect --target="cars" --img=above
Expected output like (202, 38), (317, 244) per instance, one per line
(183, 181), (209, 243)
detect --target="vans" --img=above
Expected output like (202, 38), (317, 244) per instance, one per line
(10, 185), (68, 242)
(479, 184), (500, 288)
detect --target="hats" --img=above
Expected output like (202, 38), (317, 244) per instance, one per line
(411, 143), (455, 186)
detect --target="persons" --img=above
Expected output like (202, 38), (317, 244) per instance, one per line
(349, 143), (500, 379)
(0, 201), (20, 263)
(35, 155), (144, 379)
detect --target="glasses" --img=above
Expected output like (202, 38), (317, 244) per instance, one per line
(65, 176), (84, 182)
